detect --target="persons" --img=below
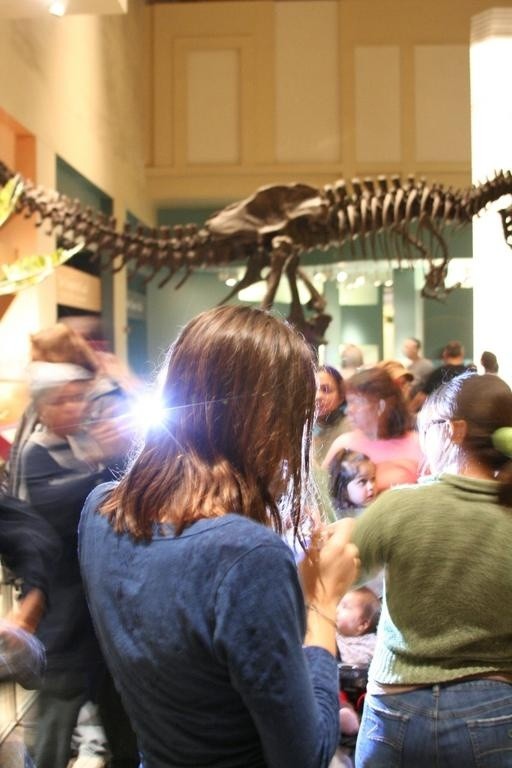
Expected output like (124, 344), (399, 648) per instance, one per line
(1, 303), (511, 768)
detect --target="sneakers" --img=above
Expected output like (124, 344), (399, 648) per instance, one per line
(71, 748), (108, 767)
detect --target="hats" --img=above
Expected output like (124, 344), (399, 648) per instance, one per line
(26, 362), (94, 388)
(377, 359), (415, 383)
